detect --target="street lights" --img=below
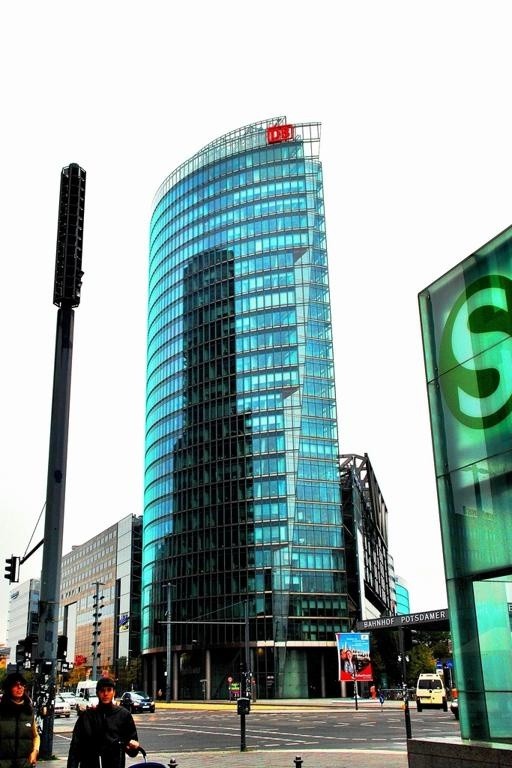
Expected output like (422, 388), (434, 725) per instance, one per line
(162, 582), (176, 702)
(91, 579), (106, 680)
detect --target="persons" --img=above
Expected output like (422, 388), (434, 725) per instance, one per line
(340, 649), (349, 672)
(0, 671), (42, 768)
(343, 650), (356, 673)
(67, 677), (141, 768)
(158, 689), (163, 701)
(369, 684), (377, 700)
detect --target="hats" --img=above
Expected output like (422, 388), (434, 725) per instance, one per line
(97, 678), (114, 689)
(5, 674), (26, 686)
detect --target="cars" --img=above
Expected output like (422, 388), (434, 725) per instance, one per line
(49, 681), (156, 718)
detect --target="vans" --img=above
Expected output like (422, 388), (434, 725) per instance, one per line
(415, 672), (447, 713)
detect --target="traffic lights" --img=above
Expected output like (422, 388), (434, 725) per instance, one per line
(4, 556), (17, 583)
(356, 660), (362, 672)
(402, 627), (418, 651)
